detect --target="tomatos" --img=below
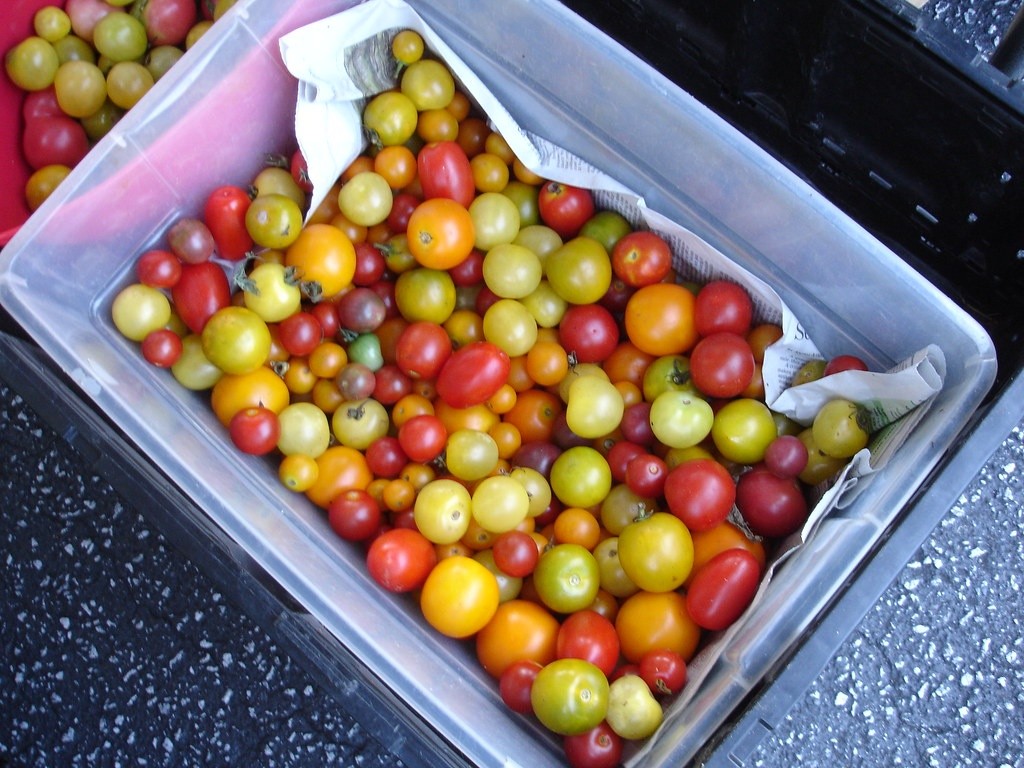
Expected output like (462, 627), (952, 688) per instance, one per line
(110, 28), (871, 768)
(6, 0), (239, 217)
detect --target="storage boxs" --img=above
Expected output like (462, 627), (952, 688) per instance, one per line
(0, 0), (1000, 768)
